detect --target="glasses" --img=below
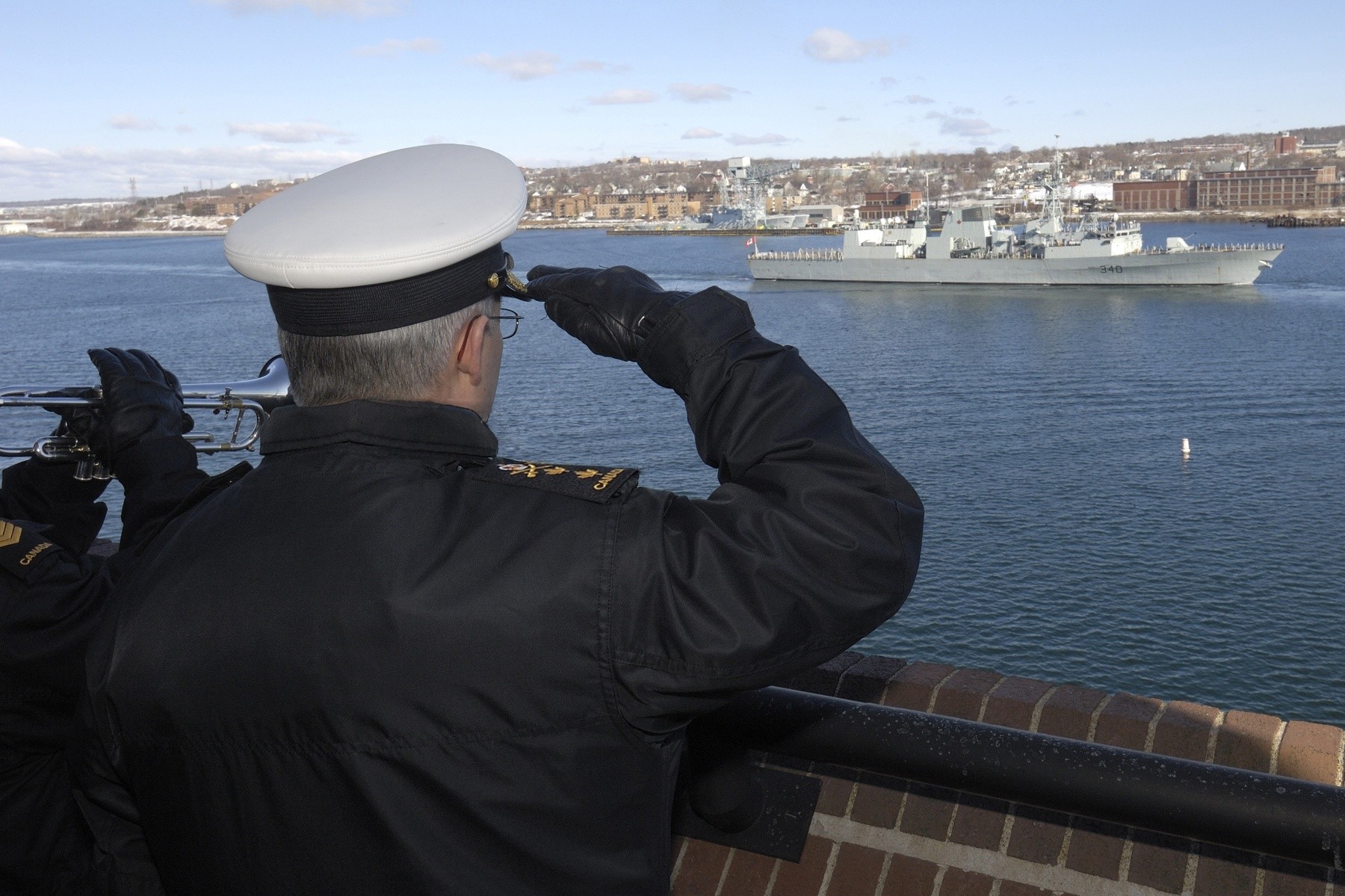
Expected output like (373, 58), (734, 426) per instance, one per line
(458, 308), (523, 365)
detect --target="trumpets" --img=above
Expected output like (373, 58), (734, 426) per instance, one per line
(2, 353), (297, 482)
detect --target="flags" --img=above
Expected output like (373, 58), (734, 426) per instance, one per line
(745, 234), (755, 247)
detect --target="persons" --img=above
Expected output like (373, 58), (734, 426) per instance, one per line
(0, 142), (925, 896)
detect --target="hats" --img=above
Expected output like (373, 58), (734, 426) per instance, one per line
(224, 144), (531, 336)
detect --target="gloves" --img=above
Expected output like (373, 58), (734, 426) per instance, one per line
(27, 420), (112, 504)
(40, 346), (195, 496)
(523, 265), (696, 363)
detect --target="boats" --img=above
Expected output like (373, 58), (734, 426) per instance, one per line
(744, 184), (1287, 289)
